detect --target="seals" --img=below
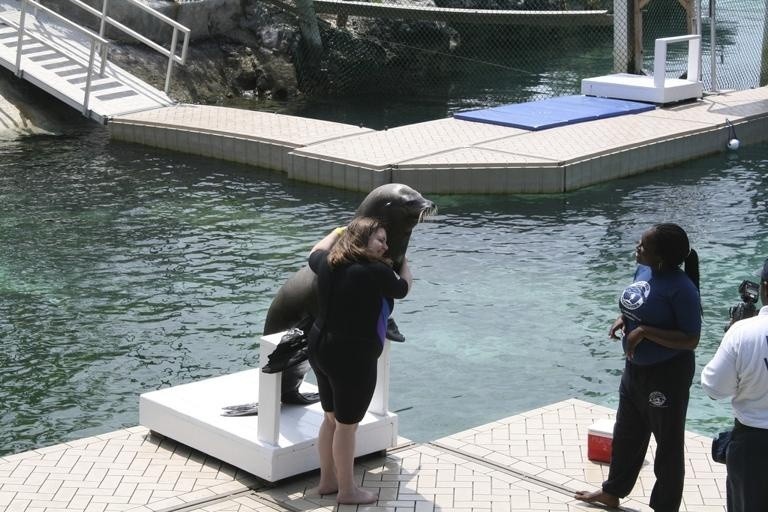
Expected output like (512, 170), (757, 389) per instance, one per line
(221, 181), (440, 419)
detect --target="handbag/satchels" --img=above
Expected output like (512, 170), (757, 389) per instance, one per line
(710, 429), (735, 465)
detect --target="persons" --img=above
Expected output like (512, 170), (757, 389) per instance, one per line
(574, 221), (703, 512)
(701, 256), (768, 511)
(305, 215), (412, 505)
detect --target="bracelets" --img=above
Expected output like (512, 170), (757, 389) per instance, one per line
(334, 226), (344, 236)
